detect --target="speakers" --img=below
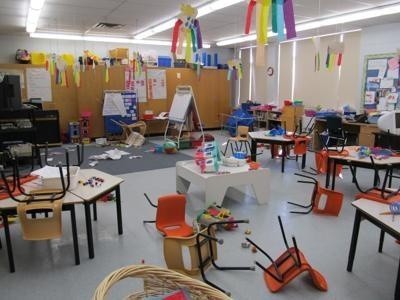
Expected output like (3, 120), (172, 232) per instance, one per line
(32, 110), (60, 147)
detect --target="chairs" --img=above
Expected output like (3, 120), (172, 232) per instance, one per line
(289, 172), (343, 217)
(347, 155), (400, 204)
(307, 132), (342, 176)
(325, 116), (347, 146)
(93, 264), (234, 300)
(245, 216), (327, 290)
(163, 219), (255, 297)
(111, 118), (146, 144)
(0, 158), (65, 241)
(222, 126), (250, 156)
(143, 190), (194, 237)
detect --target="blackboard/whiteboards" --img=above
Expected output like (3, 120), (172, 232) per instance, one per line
(170, 91), (192, 122)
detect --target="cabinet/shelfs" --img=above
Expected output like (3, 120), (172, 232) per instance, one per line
(1, 64), (231, 139)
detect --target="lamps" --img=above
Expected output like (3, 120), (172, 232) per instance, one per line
(216, 0), (400, 48)
(26, 0), (44, 34)
(132, 0), (245, 41)
(28, 32), (215, 48)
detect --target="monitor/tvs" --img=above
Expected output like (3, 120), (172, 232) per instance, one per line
(0, 75), (21, 109)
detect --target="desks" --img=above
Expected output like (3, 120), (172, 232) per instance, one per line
(346, 190), (400, 300)
(313, 119), (380, 148)
(0, 168), (124, 273)
(319, 147), (400, 191)
(248, 130), (307, 173)
